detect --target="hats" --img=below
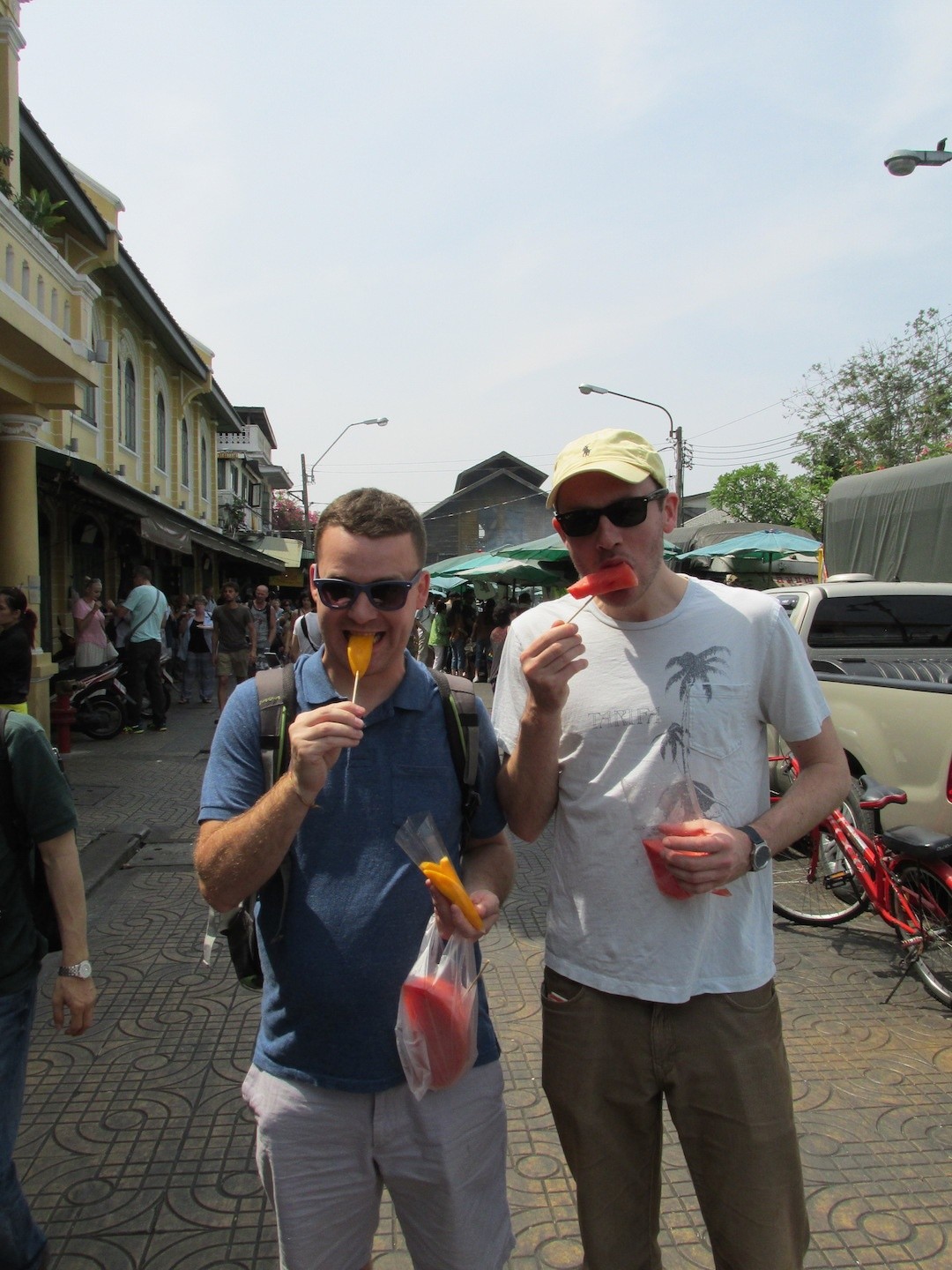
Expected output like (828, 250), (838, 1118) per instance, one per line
(545, 430), (668, 510)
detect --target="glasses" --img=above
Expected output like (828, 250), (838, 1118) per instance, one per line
(552, 489), (669, 539)
(312, 558), (422, 610)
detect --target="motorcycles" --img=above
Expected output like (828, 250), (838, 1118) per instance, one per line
(50, 647), (179, 740)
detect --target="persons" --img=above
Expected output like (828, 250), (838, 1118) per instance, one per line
(491, 429), (850, 1270)
(195, 487), (515, 1270)
(407, 591), (532, 690)
(76, 565), (321, 734)
(0, 584), (97, 1270)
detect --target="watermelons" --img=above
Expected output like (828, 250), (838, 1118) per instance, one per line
(404, 975), (472, 1087)
(566, 562), (638, 599)
(643, 838), (711, 899)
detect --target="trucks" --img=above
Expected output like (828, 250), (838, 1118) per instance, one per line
(821, 454), (952, 582)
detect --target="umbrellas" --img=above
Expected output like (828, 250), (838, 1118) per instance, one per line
(675, 529), (823, 588)
(424, 530), (683, 597)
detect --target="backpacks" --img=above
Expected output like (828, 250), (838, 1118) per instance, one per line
(204, 661), (482, 994)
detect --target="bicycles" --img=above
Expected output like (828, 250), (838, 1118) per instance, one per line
(770, 753), (952, 1011)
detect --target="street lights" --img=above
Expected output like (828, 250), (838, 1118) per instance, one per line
(578, 384), (684, 525)
(300, 417), (388, 550)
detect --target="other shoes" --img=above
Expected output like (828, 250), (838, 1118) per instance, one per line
(177, 696), (191, 703)
(204, 697), (212, 702)
(473, 676), (481, 683)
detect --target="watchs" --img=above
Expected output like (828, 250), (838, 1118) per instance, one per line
(738, 826), (770, 871)
(60, 960), (92, 979)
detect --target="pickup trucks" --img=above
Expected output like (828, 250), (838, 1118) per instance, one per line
(761, 571), (952, 841)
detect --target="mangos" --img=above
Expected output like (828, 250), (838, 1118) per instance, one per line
(418, 856), (484, 932)
(347, 634), (374, 677)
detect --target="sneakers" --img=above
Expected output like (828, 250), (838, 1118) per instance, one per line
(147, 721), (168, 732)
(122, 726), (143, 734)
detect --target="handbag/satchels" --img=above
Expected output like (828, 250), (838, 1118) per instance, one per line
(106, 641), (119, 662)
(123, 629), (134, 645)
(393, 908), (480, 1100)
